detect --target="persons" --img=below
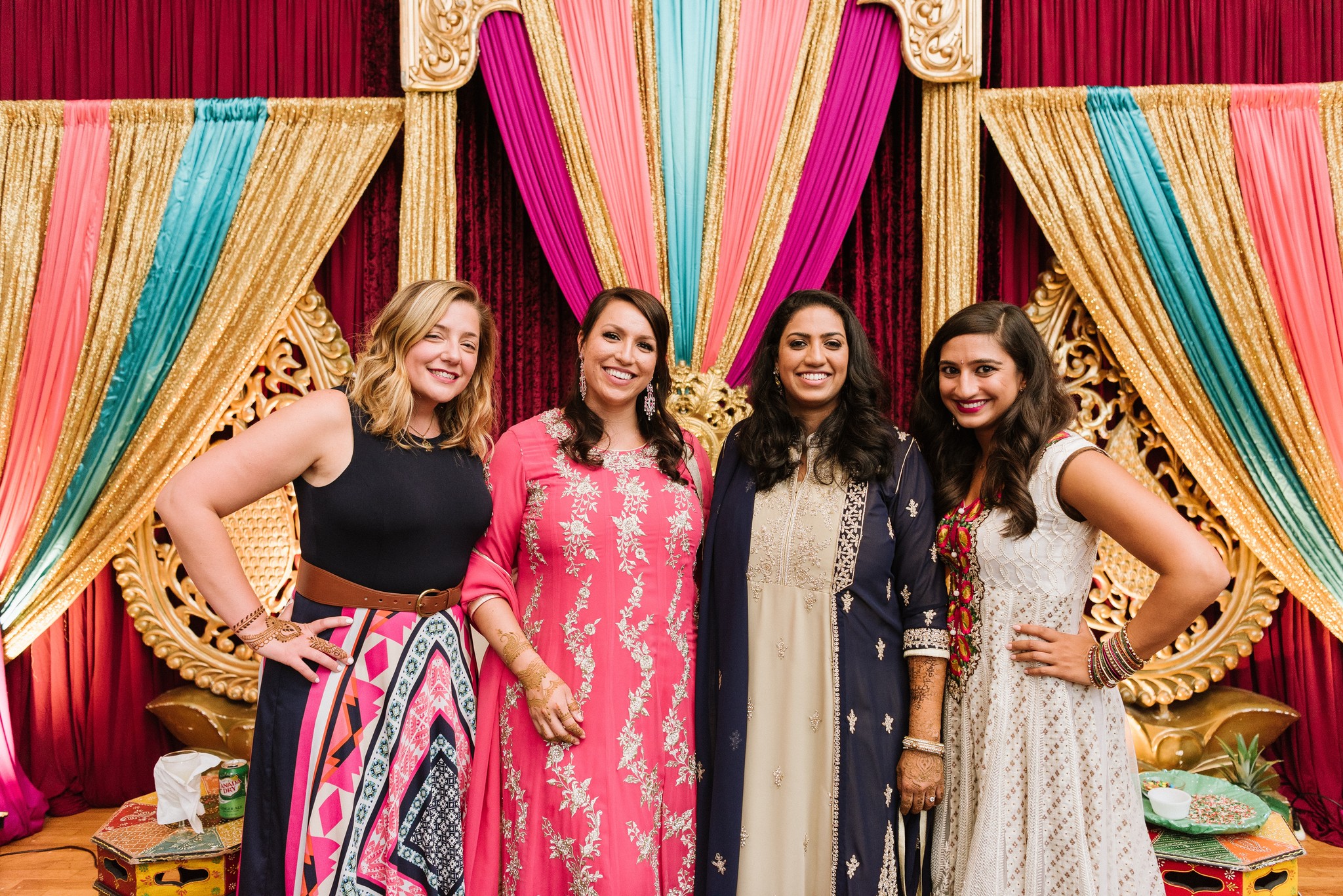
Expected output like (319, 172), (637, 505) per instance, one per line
(694, 290), (952, 896)
(154, 279), (497, 896)
(460, 287), (712, 896)
(914, 302), (1228, 896)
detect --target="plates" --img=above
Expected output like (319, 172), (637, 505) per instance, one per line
(1138, 769), (1272, 834)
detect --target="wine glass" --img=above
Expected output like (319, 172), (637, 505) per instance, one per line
(161, 750), (202, 842)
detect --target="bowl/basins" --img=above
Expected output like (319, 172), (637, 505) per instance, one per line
(1139, 773), (1186, 799)
(1148, 787), (1191, 820)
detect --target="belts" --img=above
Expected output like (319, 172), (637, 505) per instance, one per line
(295, 556), (465, 617)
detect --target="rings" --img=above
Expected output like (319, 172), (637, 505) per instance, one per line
(925, 794), (936, 802)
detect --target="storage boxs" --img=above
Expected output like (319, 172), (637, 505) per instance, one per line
(90, 775), (246, 896)
(1144, 810), (1307, 896)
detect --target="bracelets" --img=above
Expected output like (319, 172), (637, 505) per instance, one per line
(1088, 621), (1151, 691)
(902, 736), (945, 759)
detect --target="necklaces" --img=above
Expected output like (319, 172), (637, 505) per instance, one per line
(978, 459), (987, 469)
(406, 408), (434, 452)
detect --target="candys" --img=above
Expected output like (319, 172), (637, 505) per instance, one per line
(1143, 780), (1177, 790)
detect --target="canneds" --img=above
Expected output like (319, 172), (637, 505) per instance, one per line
(217, 759), (250, 819)
